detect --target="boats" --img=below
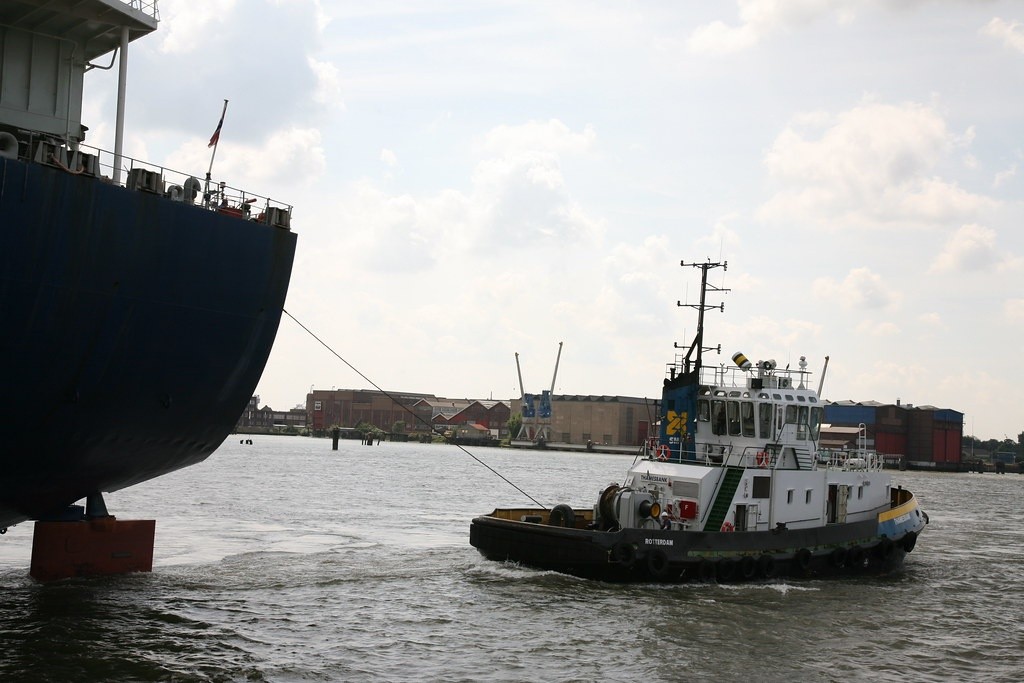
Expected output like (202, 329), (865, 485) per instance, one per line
(469, 236), (930, 589)
(0, 0), (298, 585)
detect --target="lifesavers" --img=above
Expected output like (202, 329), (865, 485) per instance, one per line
(167, 184), (185, 203)
(548, 502), (576, 528)
(614, 540), (636, 568)
(656, 444), (671, 460)
(754, 453), (768, 469)
(720, 522), (734, 532)
(695, 555), (777, 584)
(830, 531), (916, 572)
(794, 547), (814, 577)
(647, 547), (670, 576)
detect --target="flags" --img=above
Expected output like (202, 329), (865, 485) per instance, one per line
(208, 103), (228, 147)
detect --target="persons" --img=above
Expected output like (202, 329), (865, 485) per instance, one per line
(660, 512), (674, 530)
